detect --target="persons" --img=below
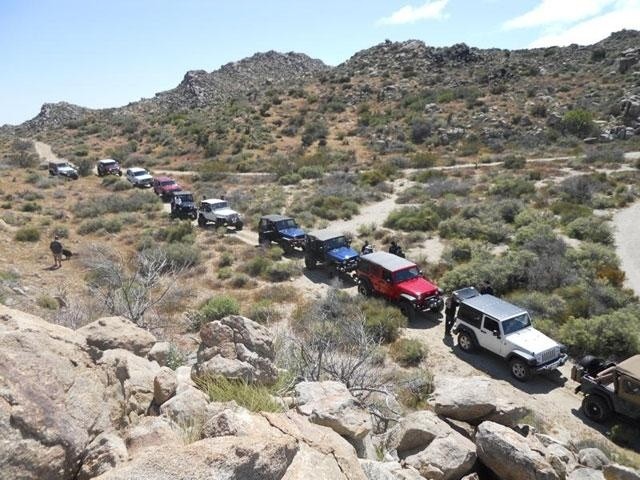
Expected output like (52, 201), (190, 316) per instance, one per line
(361, 240), (373, 254)
(389, 241), (398, 255)
(50, 235), (64, 267)
(364, 245), (372, 254)
(396, 246), (405, 258)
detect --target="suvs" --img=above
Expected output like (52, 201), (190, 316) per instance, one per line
(356, 251), (445, 321)
(97, 159), (122, 178)
(448, 285), (568, 382)
(169, 190), (197, 220)
(258, 215), (307, 254)
(306, 229), (361, 278)
(48, 160), (78, 179)
(153, 177), (181, 196)
(198, 198), (242, 233)
(125, 167), (154, 186)
(571, 353), (640, 426)
